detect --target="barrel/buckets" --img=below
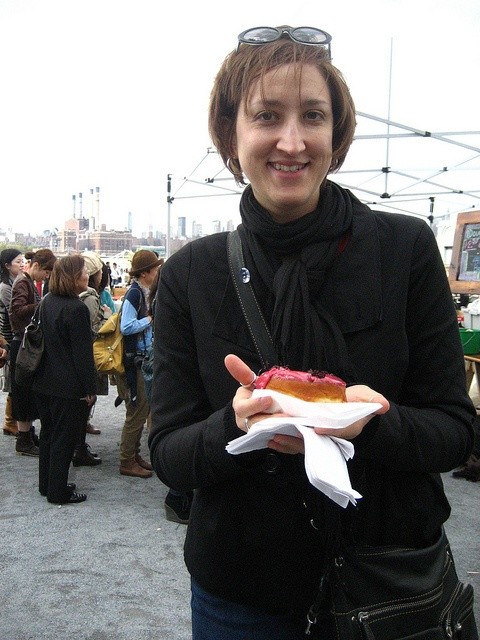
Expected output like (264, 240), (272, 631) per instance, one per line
(459, 327), (480, 354)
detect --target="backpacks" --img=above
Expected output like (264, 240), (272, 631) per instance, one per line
(92, 289), (143, 375)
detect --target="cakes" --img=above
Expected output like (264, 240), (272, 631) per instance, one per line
(254, 361), (348, 405)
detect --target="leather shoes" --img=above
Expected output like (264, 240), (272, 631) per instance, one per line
(137, 455), (152, 470)
(40, 482), (76, 496)
(87, 424), (101, 434)
(47, 492), (86, 503)
(120, 457), (153, 478)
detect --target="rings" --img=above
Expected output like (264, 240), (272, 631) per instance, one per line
(239, 371), (258, 389)
(245, 418), (251, 433)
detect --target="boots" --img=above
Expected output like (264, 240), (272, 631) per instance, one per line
(30, 425), (41, 448)
(3, 394), (18, 435)
(72, 444), (102, 468)
(15, 427), (40, 456)
(90, 450), (98, 457)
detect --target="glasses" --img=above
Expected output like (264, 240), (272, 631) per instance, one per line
(235, 26), (331, 56)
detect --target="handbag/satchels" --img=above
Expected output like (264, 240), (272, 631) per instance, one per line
(306, 525), (480, 640)
(13, 321), (45, 386)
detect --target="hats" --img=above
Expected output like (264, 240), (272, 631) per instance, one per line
(80, 251), (103, 275)
(130, 249), (164, 275)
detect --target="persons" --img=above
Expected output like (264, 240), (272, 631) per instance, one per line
(463, 238), (476, 253)
(33, 253), (98, 504)
(0, 249), (27, 437)
(1, 335), (11, 371)
(8, 248), (57, 458)
(148, 26), (480, 639)
(71, 249), (112, 468)
(114, 249), (165, 478)
(104, 260), (131, 285)
(86, 262), (117, 435)
